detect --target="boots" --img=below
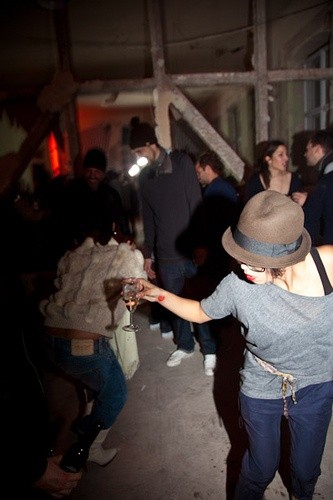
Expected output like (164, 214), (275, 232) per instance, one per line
(86, 426), (118, 467)
(84, 396), (100, 417)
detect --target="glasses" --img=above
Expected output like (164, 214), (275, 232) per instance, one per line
(237, 261), (265, 272)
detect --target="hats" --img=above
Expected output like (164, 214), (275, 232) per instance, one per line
(222, 190), (312, 269)
(129, 122), (157, 149)
(84, 147), (107, 173)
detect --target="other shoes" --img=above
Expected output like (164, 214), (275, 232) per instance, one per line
(162, 330), (173, 339)
(166, 350), (194, 368)
(204, 354), (216, 376)
(149, 323), (160, 331)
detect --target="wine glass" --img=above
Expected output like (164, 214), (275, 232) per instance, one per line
(120, 280), (142, 332)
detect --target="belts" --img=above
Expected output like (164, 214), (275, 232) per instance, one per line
(50, 328), (104, 339)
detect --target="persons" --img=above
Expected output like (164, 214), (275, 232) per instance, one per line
(120, 190), (333, 500)
(0, 124), (333, 500)
